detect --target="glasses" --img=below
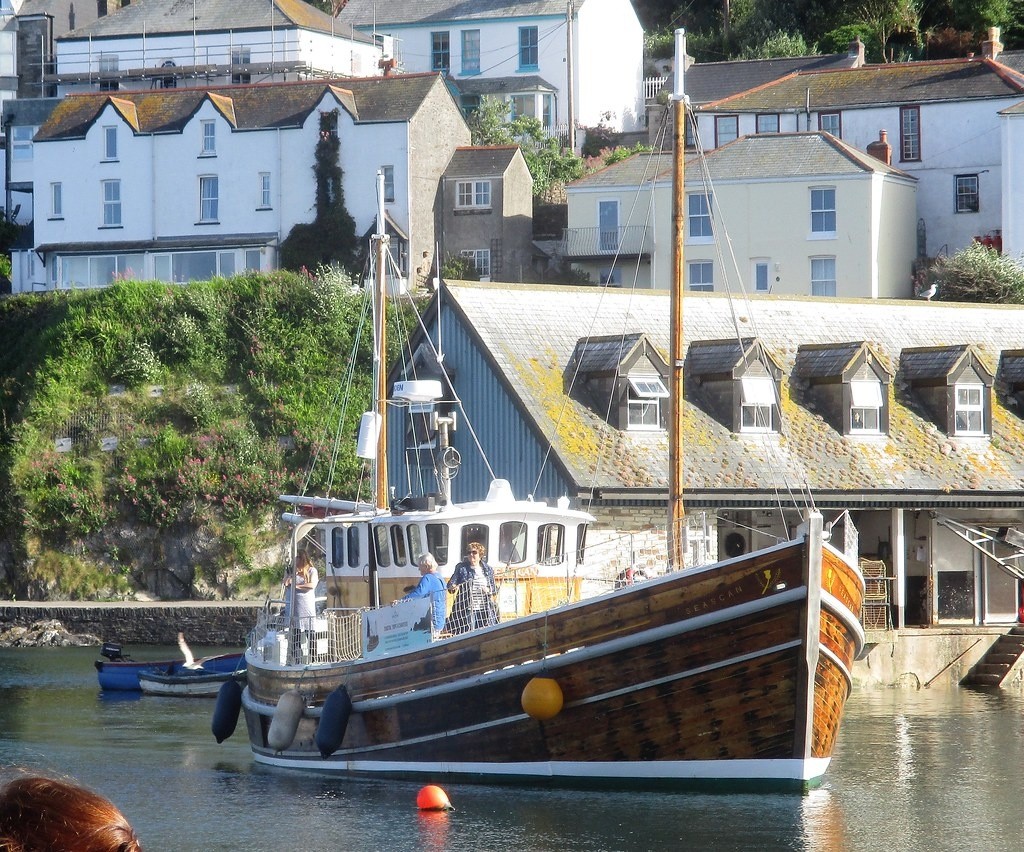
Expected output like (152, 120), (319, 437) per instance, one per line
(468, 551), (479, 555)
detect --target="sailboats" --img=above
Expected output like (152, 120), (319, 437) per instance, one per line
(245, 28), (867, 793)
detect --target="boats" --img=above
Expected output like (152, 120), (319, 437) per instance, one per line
(93, 640), (247, 697)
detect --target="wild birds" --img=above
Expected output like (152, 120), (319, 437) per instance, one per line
(178, 632), (229, 671)
(919, 284), (938, 302)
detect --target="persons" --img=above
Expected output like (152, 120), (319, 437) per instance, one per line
(283, 549), (320, 665)
(447, 542), (500, 635)
(390, 553), (446, 642)
(0, 771), (143, 852)
